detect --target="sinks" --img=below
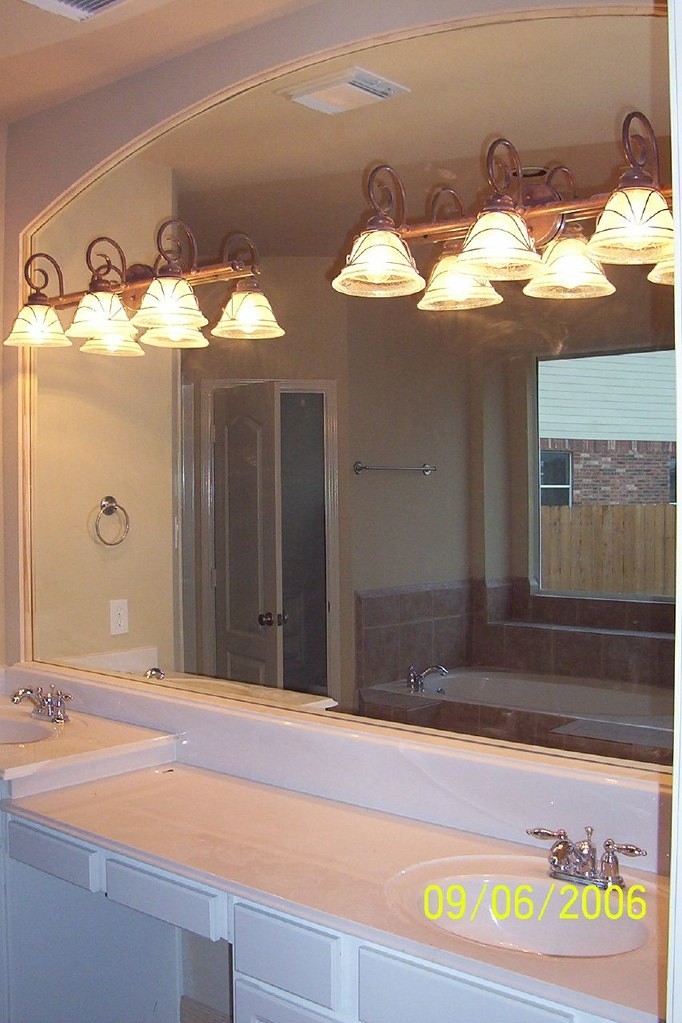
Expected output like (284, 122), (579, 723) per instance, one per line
(154, 678), (252, 696)
(389, 849), (669, 969)
(0, 704), (84, 750)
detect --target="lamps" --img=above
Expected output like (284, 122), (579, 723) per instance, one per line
(55, 231), (284, 357)
(3, 218), (245, 347)
(418, 164), (675, 313)
(330, 110), (674, 299)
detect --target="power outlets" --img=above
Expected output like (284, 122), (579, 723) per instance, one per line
(108, 598), (129, 635)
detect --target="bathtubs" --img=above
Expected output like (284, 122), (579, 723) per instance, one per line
(358, 667), (673, 770)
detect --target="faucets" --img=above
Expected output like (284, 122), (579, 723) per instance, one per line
(14, 688), (55, 719)
(145, 667), (163, 681)
(549, 825), (597, 879)
(416, 664), (449, 690)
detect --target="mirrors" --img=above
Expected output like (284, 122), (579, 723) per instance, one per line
(15, 5), (676, 779)
